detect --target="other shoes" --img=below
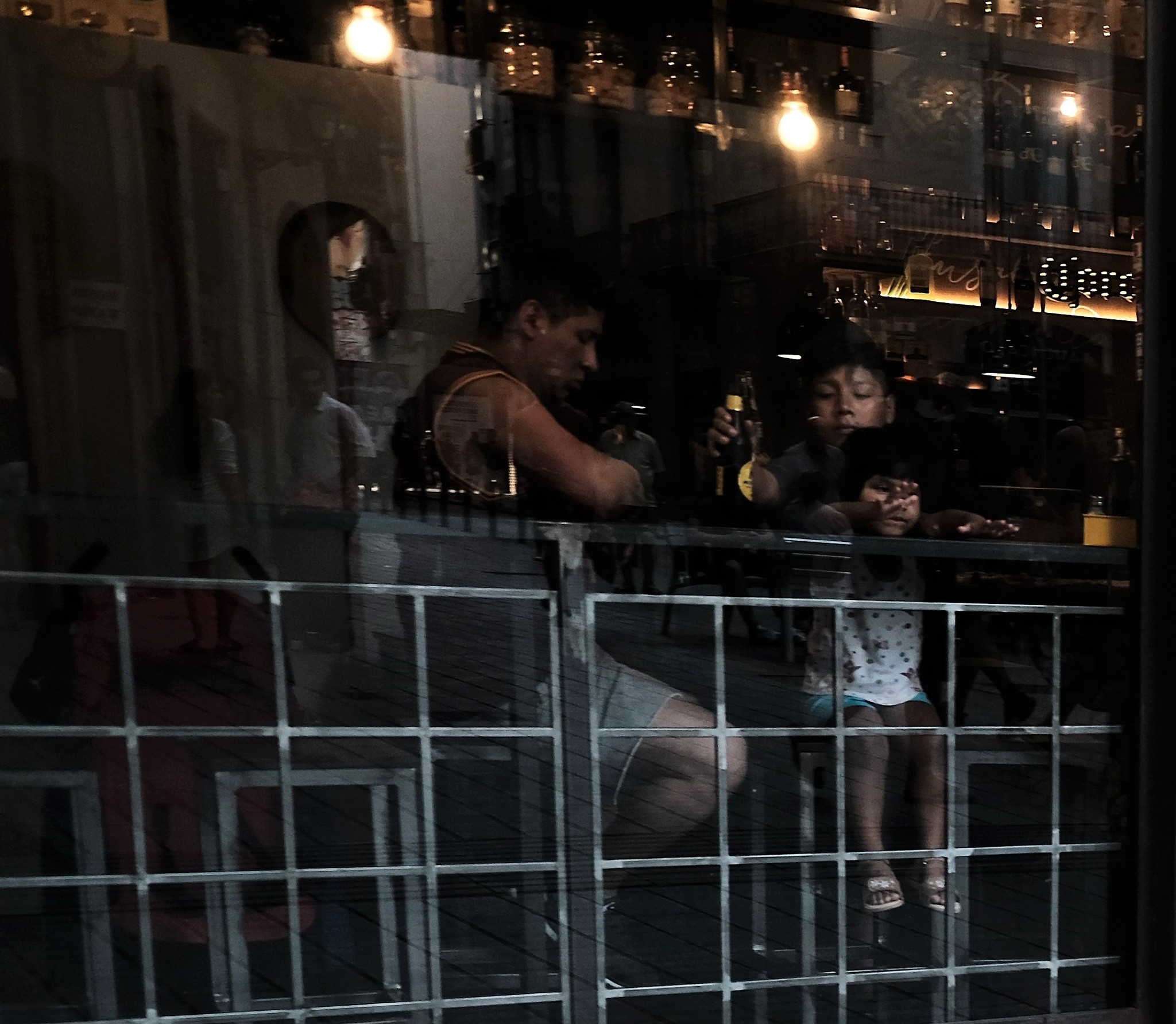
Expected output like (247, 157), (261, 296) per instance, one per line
(542, 892), (662, 989)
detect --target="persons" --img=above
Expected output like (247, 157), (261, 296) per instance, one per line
(542, 383), (809, 658)
(390, 247), (751, 995)
(151, 350), (379, 722)
(54, 468), (330, 1014)
(711, 340), (899, 528)
(794, 423), (1023, 909)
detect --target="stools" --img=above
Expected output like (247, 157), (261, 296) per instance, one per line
(794, 737), (1125, 1024)
(0, 752), (118, 1021)
(190, 717), (766, 1024)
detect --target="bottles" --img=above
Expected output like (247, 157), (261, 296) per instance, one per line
(493, 1), (860, 119)
(1086, 495), (1105, 515)
(821, 272), (887, 357)
(961, 0), (1147, 205)
(906, 240), (933, 300)
(1013, 249), (1035, 312)
(905, 348), (929, 378)
(713, 385), (753, 505)
(976, 239), (997, 307)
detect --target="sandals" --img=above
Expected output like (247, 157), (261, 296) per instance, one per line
(859, 858), (904, 912)
(922, 857), (962, 914)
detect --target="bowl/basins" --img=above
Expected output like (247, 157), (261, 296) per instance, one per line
(1082, 513), (1137, 548)
